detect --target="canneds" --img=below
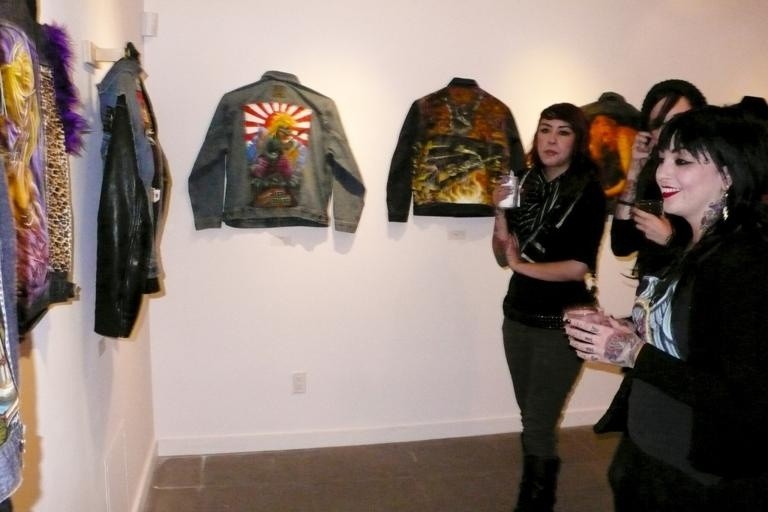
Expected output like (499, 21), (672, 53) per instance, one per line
(499, 175), (518, 210)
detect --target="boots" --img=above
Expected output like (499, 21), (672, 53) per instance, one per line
(514, 456), (558, 512)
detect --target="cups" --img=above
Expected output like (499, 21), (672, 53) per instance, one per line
(635, 197), (660, 216)
(564, 305), (594, 329)
(496, 172), (518, 211)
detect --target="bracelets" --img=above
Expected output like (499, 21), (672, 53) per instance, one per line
(618, 199), (635, 207)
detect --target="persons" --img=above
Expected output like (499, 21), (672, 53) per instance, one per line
(562, 96), (768, 511)
(492, 102), (609, 512)
(610, 78), (709, 285)
